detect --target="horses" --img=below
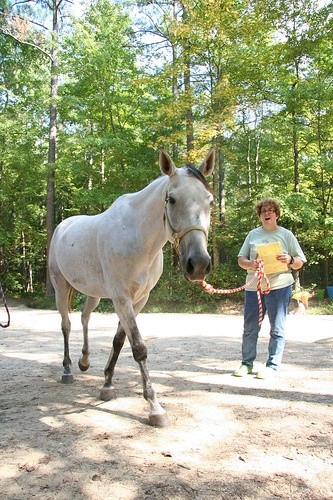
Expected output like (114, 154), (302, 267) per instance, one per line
(48, 145), (216, 428)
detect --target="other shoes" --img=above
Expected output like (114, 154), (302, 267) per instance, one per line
(234, 365), (252, 377)
(260, 210), (276, 214)
(256, 367), (278, 379)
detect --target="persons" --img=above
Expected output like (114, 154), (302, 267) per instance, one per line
(288, 291), (314, 316)
(233, 199), (307, 379)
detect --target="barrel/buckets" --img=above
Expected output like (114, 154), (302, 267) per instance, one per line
(326, 286), (333, 298)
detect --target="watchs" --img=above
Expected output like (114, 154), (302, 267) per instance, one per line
(288, 256), (295, 265)
